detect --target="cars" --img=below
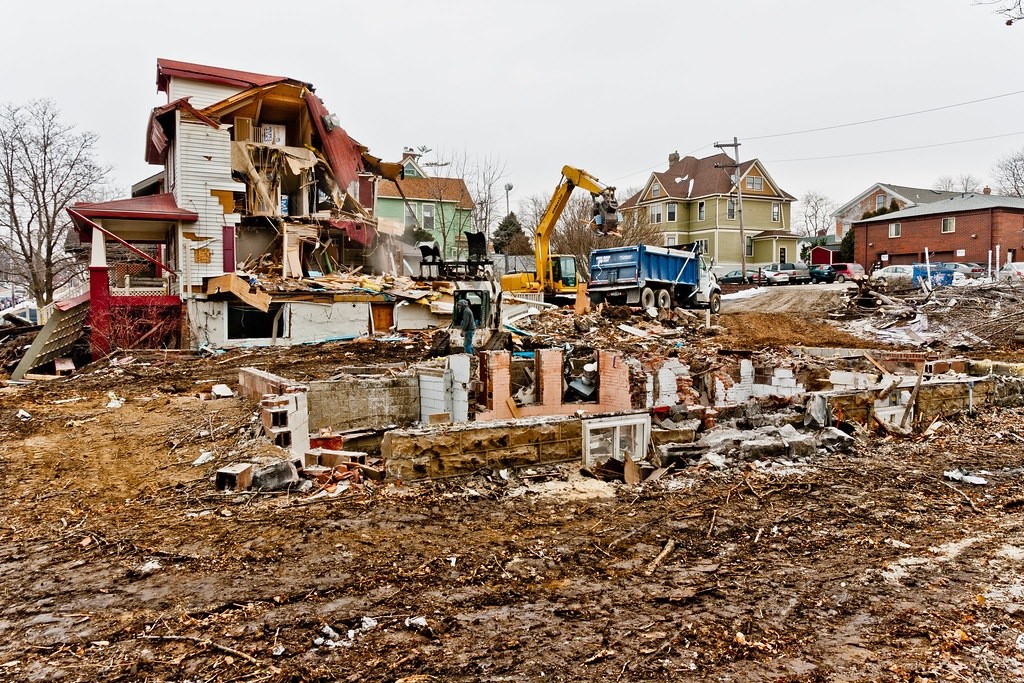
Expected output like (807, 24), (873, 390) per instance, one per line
(870, 261), (1024, 287)
(718, 262), (866, 286)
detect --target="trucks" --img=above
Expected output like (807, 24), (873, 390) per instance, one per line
(587, 241), (722, 315)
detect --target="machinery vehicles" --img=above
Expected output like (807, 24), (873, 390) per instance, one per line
(498, 164), (621, 308)
(423, 289), (513, 358)
